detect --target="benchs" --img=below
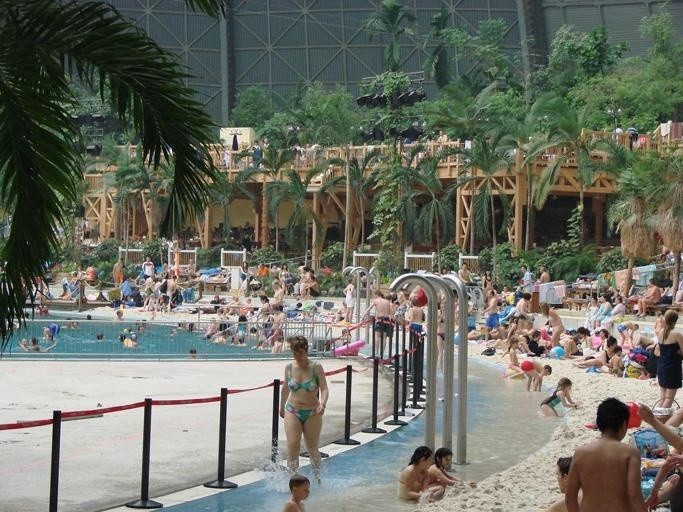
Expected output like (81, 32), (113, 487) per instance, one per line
(563, 297), (588, 311)
(642, 304), (669, 317)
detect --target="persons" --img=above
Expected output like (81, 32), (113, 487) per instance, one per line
(79, 120), (675, 187)
(281, 473), (310, 512)
(424, 447), (479, 490)
(544, 455), (584, 511)
(536, 378), (578, 418)
(2, 217), (682, 391)
(652, 308), (681, 410)
(564, 398), (649, 512)
(394, 446), (445, 503)
(632, 401), (682, 511)
(280, 335), (329, 483)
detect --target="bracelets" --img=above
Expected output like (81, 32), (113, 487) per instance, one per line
(319, 403), (325, 409)
